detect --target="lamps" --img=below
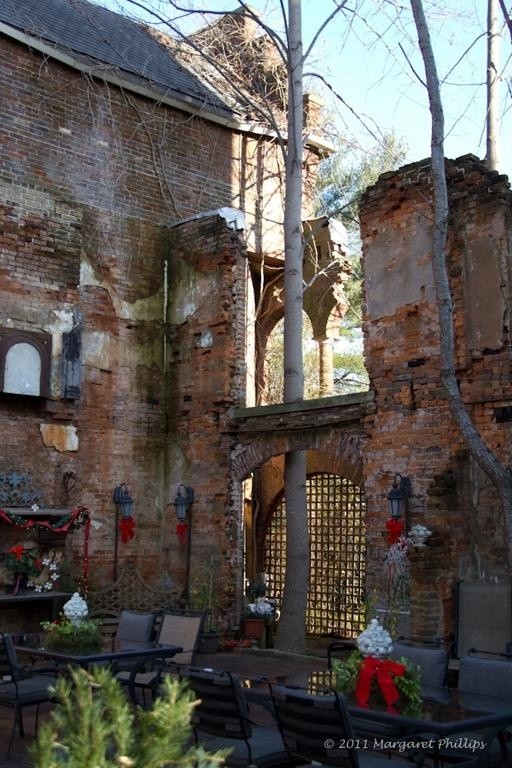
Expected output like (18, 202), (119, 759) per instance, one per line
(173, 482), (194, 524)
(111, 481), (134, 520)
(387, 470), (413, 521)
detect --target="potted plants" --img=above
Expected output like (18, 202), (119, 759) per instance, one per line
(188, 559), (224, 652)
(383, 536), (412, 612)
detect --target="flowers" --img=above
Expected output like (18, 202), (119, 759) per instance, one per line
(26, 557), (60, 593)
(3, 540), (43, 579)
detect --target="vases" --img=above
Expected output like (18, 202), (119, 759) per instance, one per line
(9, 575), (25, 596)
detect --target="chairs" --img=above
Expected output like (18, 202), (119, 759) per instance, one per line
(0, 609), (512, 768)
